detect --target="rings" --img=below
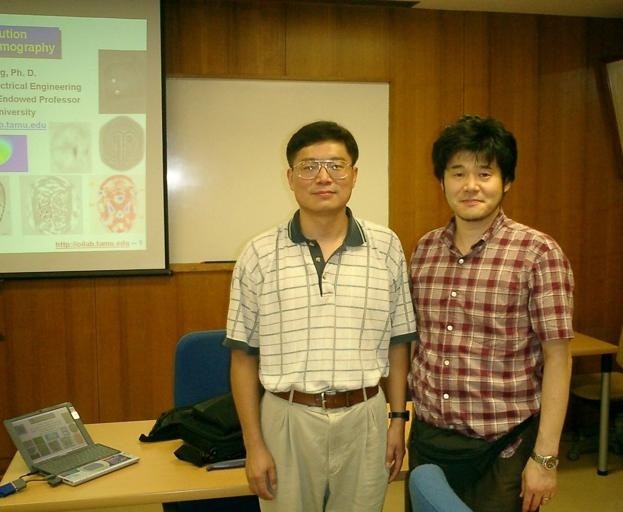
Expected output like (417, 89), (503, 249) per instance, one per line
(541, 495), (552, 500)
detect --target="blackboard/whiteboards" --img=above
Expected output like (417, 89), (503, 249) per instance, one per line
(157, 72), (396, 274)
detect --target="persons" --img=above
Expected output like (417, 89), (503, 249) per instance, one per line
(398, 115), (579, 510)
(223, 114), (417, 509)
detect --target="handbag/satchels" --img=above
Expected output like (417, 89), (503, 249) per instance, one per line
(409, 419), (497, 485)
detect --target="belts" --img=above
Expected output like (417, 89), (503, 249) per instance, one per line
(271, 385), (378, 408)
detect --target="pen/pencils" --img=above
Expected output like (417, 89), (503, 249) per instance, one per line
(201, 262), (237, 264)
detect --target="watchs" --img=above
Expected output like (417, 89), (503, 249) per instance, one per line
(529, 448), (561, 472)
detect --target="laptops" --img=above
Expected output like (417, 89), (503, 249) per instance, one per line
(3, 401), (140, 486)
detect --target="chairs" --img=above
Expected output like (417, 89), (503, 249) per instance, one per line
(409, 461), (473, 512)
(174, 330), (231, 407)
(572, 333), (623, 463)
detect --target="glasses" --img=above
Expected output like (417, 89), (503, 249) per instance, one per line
(294, 160), (352, 179)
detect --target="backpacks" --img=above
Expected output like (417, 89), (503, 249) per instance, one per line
(139, 393), (246, 466)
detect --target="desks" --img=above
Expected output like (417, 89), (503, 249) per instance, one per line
(570, 329), (619, 476)
(0, 400), (416, 512)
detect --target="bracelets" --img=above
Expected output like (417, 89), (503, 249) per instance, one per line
(387, 409), (411, 423)
